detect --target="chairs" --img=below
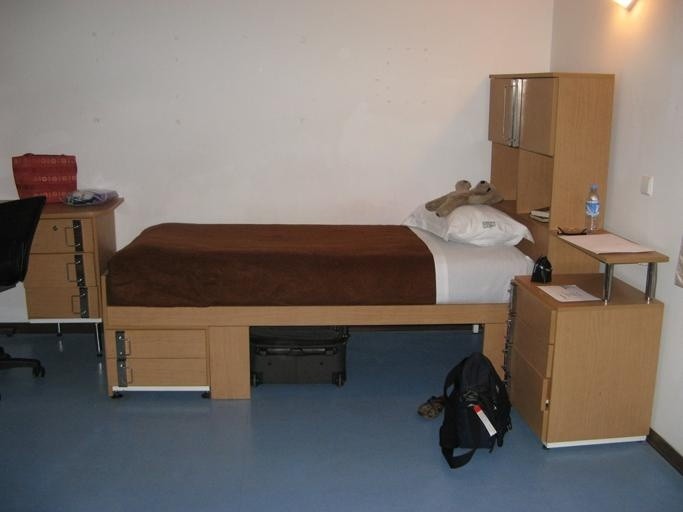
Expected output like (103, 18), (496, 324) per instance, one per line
(0, 193), (47, 379)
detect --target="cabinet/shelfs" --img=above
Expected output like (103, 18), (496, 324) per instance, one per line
(24, 197), (123, 319)
(490, 73), (615, 271)
(503, 273), (666, 449)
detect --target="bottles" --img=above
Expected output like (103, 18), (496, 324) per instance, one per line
(584, 183), (601, 233)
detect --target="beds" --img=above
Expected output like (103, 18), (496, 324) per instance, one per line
(104, 223), (541, 401)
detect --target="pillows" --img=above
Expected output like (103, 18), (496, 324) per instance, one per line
(402, 203), (537, 249)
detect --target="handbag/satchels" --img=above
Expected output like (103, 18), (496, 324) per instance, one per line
(439, 350), (511, 467)
(12, 152), (77, 205)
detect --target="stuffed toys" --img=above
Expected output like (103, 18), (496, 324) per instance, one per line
(426, 180), (504, 217)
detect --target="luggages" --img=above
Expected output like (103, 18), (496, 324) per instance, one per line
(251, 327), (351, 388)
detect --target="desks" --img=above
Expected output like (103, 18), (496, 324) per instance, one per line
(552, 229), (671, 304)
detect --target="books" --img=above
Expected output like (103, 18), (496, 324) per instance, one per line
(529, 207), (551, 223)
